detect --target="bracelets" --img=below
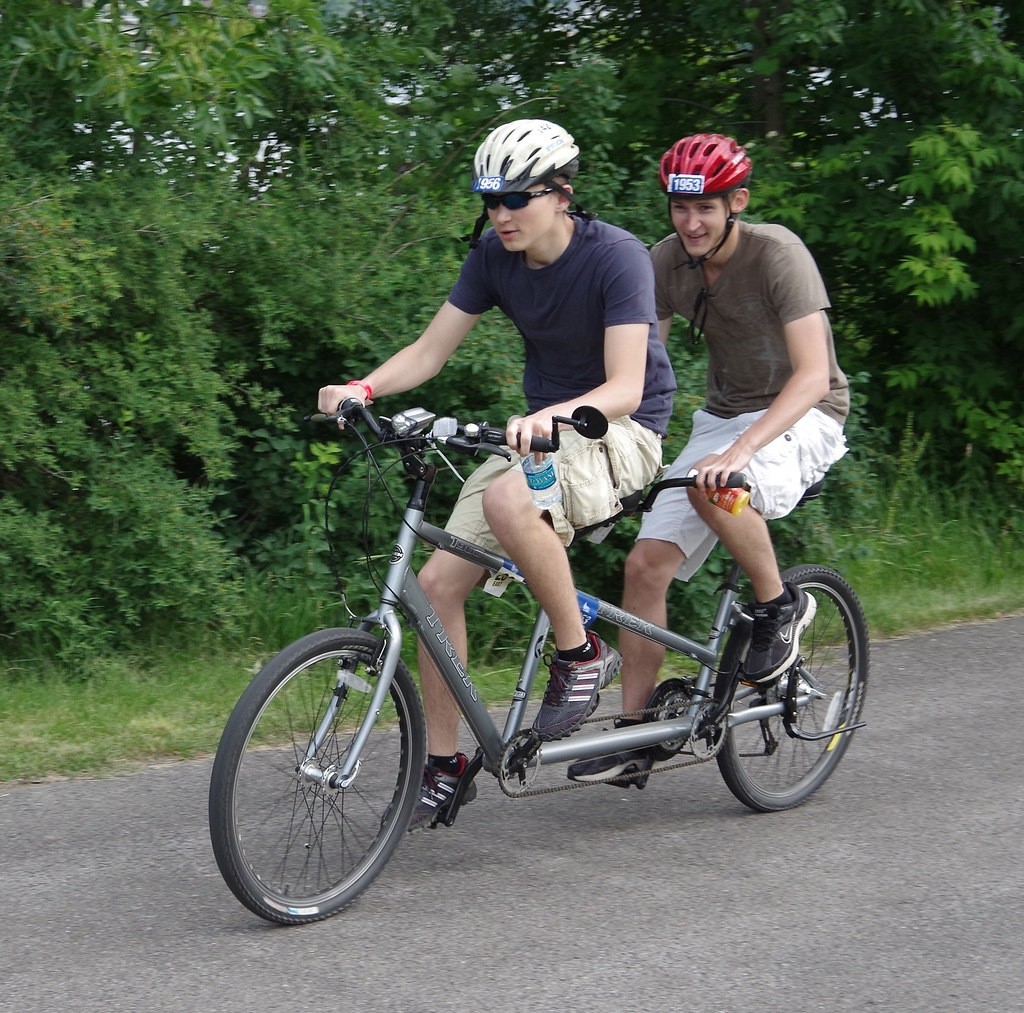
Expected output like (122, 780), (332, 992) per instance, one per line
(345, 380), (371, 401)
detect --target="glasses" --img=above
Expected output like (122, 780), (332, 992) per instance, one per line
(481, 188), (555, 209)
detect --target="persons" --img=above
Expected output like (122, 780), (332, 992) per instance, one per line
(317, 119), (678, 837)
(565, 132), (851, 785)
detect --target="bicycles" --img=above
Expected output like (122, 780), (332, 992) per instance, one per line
(206, 396), (871, 927)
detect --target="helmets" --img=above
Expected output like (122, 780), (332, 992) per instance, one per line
(660, 133), (753, 200)
(472, 120), (579, 193)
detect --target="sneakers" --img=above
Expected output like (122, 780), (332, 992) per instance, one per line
(533, 630), (624, 741)
(406, 753), (477, 836)
(743, 582), (817, 683)
(567, 718), (651, 781)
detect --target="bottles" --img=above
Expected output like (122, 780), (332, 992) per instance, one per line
(521, 452), (562, 509)
(687, 468), (750, 515)
